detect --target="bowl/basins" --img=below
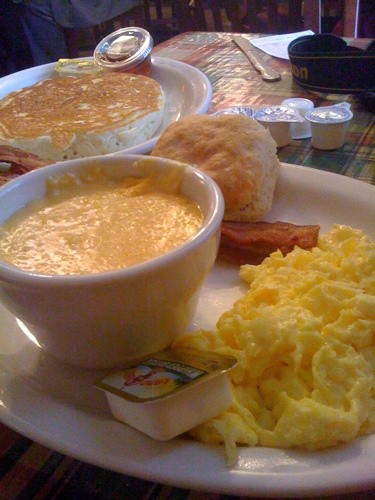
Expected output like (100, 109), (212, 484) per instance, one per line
(0, 154), (226, 371)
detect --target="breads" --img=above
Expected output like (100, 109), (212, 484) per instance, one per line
(149, 112), (278, 222)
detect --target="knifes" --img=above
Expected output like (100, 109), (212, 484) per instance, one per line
(232, 35), (281, 82)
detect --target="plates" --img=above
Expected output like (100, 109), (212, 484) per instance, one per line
(0, 161), (375, 498)
(0, 55), (211, 165)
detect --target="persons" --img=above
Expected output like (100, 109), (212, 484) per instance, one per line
(0, 0), (151, 77)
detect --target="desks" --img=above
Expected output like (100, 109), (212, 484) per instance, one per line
(0, 32), (375, 500)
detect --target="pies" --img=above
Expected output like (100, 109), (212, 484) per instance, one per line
(0, 72), (164, 162)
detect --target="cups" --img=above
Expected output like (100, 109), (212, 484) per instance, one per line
(255, 105), (306, 148)
(93, 26), (155, 77)
(281, 97), (315, 140)
(305, 101), (354, 151)
